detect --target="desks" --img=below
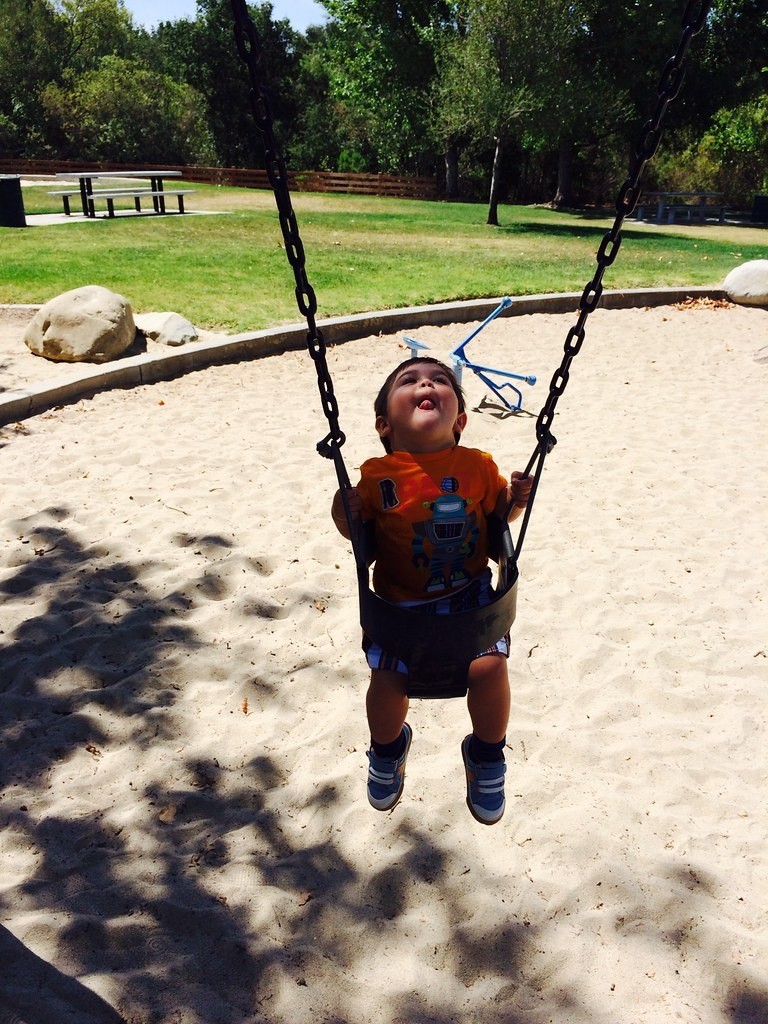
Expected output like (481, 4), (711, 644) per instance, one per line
(56, 170), (182, 216)
(646, 192), (725, 224)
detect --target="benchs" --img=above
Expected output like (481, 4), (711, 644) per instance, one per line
(664, 205), (732, 224)
(633, 204), (698, 221)
(47, 187), (152, 217)
(88, 189), (198, 217)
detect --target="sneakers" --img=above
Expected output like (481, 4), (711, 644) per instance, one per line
(461, 734), (507, 825)
(365, 722), (412, 811)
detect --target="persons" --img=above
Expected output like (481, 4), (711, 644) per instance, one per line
(331, 356), (533, 824)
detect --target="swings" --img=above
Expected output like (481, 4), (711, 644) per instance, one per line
(228, 0), (711, 699)
